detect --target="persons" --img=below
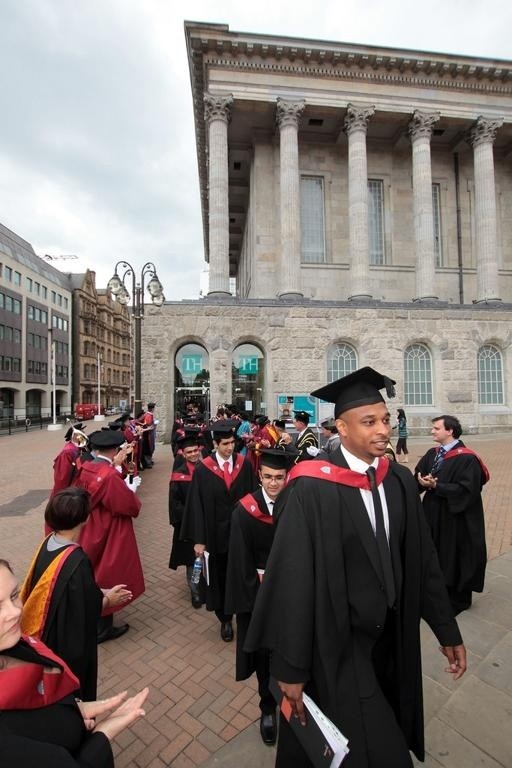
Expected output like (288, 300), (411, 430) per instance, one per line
(169, 406), (342, 745)
(385, 409), (409, 465)
(415, 415), (488, 614)
(240, 365), (468, 768)
(2, 558), (149, 768)
(18, 486), (135, 725)
(45, 405), (155, 642)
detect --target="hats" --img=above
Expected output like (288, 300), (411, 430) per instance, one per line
(292, 410), (314, 423)
(310, 365), (397, 420)
(251, 447), (302, 469)
(175, 435), (200, 449)
(64, 422), (87, 442)
(204, 419), (241, 440)
(90, 430), (125, 448)
(273, 420), (287, 427)
(87, 412), (130, 439)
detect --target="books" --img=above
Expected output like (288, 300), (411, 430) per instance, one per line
(263, 672), (351, 768)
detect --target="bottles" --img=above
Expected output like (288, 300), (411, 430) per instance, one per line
(191, 555), (202, 584)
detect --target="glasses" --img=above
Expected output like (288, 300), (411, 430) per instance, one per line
(264, 474), (284, 480)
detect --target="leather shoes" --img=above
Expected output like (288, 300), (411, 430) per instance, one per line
(221, 621), (233, 642)
(192, 594), (201, 608)
(260, 709), (277, 745)
(108, 623), (129, 639)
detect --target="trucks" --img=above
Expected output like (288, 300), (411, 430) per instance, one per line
(74, 403), (106, 421)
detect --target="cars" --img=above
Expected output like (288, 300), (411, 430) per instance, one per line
(104, 406), (120, 415)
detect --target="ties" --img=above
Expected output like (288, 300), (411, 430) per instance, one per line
(224, 461), (232, 490)
(430, 447), (445, 475)
(366, 465), (396, 608)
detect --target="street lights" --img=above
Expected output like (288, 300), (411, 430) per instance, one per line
(95, 342), (101, 414)
(108, 261), (165, 471)
(46, 327), (57, 424)
